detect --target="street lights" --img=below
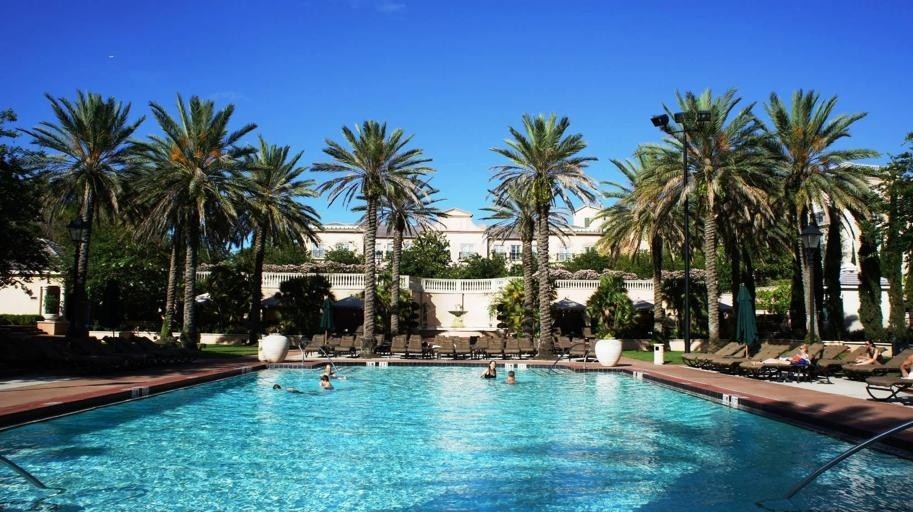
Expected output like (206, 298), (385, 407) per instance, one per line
(798, 200), (821, 347)
(64, 213), (93, 374)
(649, 109), (712, 351)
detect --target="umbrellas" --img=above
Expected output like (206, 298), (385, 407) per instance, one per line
(320, 296), (333, 345)
(734, 280), (760, 359)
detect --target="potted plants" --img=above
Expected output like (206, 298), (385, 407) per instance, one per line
(588, 274), (634, 368)
(262, 293), (291, 363)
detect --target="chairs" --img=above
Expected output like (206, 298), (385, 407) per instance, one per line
(300, 330), (594, 359)
(682, 339), (913, 406)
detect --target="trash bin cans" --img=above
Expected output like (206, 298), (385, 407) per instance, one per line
(653, 343), (664, 365)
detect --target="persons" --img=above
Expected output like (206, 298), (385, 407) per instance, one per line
(855, 339), (885, 367)
(749, 343), (811, 365)
(479, 360), (497, 379)
(272, 383), (304, 394)
(319, 375), (334, 390)
(899, 354), (913, 379)
(318, 364), (336, 379)
(506, 370), (516, 384)
